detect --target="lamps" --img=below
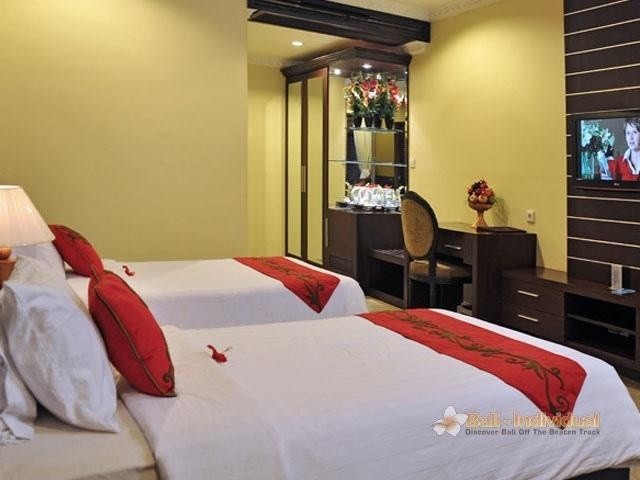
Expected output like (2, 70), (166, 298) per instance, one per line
(0, 184), (54, 286)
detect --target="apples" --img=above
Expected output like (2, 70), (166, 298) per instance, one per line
(470, 195), (478, 202)
(478, 195), (488, 203)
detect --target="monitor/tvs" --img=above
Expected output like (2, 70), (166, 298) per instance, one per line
(574, 112), (640, 190)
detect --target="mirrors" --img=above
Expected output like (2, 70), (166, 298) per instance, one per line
(345, 76), (408, 194)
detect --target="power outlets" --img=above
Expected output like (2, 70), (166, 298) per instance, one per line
(527, 210), (535, 223)
(526, 210), (535, 223)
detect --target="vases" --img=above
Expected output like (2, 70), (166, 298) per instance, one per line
(470, 202), (492, 228)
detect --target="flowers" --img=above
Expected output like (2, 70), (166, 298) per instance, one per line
(466, 181), (496, 203)
(346, 71), (402, 129)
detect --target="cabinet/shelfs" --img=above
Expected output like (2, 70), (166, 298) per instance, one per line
(503, 266), (639, 384)
(368, 248), (431, 309)
(281, 44), (410, 269)
(328, 211), (403, 301)
(436, 222), (536, 320)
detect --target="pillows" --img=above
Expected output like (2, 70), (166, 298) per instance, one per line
(47, 216), (105, 276)
(9, 242), (68, 284)
(87, 261), (181, 398)
(2, 256), (116, 436)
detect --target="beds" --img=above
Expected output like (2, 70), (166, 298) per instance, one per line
(61, 256), (368, 328)
(3, 309), (638, 480)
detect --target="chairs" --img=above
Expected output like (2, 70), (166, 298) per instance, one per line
(401, 191), (472, 309)
